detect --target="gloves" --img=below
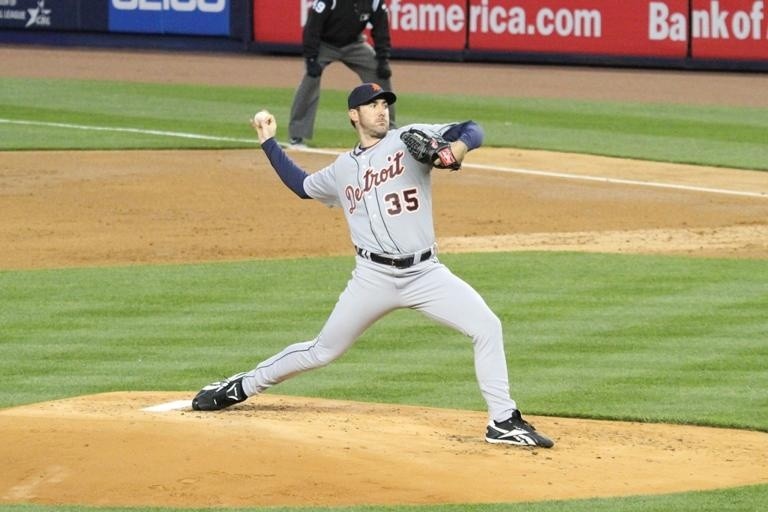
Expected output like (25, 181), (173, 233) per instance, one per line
(306, 56), (321, 77)
(376, 59), (390, 79)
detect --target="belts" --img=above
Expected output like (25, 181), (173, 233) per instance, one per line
(354, 246), (430, 268)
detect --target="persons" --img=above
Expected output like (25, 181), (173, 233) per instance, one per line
(288, 1), (398, 149)
(191, 84), (555, 448)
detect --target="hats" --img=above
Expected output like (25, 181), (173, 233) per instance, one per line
(347, 83), (396, 109)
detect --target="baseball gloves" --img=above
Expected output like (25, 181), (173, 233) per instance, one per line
(401, 129), (461, 173)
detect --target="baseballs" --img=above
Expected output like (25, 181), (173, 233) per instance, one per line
(254, 112), (270, 127)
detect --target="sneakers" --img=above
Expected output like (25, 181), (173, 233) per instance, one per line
(484, 410), (553, 448)
(288, 137), (302, 144)
(191, 372), (247, 410)
